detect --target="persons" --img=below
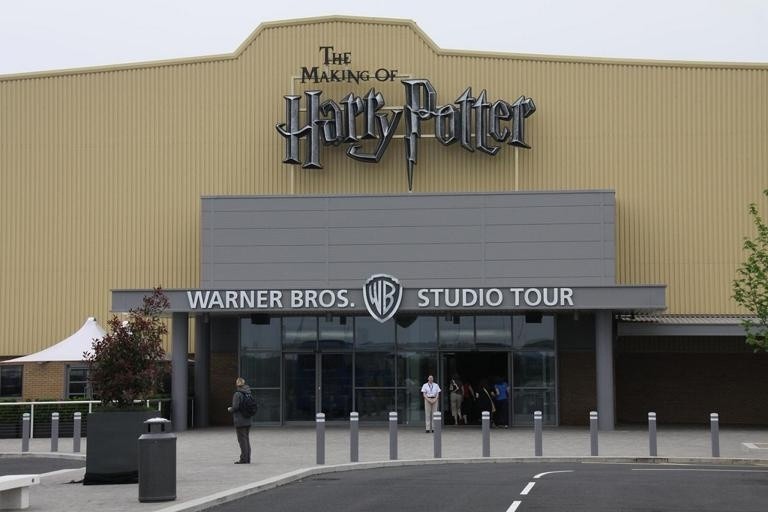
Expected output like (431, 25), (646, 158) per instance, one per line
(449, 373), (511, 429)
(421, 375), (442, 432)
(227, 377), (253, 463)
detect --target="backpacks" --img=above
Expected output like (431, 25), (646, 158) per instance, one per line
(233, 391), (257, 416)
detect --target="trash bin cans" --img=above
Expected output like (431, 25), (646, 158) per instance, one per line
(138, 417), (177, 503)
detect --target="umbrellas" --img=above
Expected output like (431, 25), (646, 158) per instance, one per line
(1, 317), (197, 400)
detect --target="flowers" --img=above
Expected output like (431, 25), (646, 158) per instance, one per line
(80, 285), (170, 406)
